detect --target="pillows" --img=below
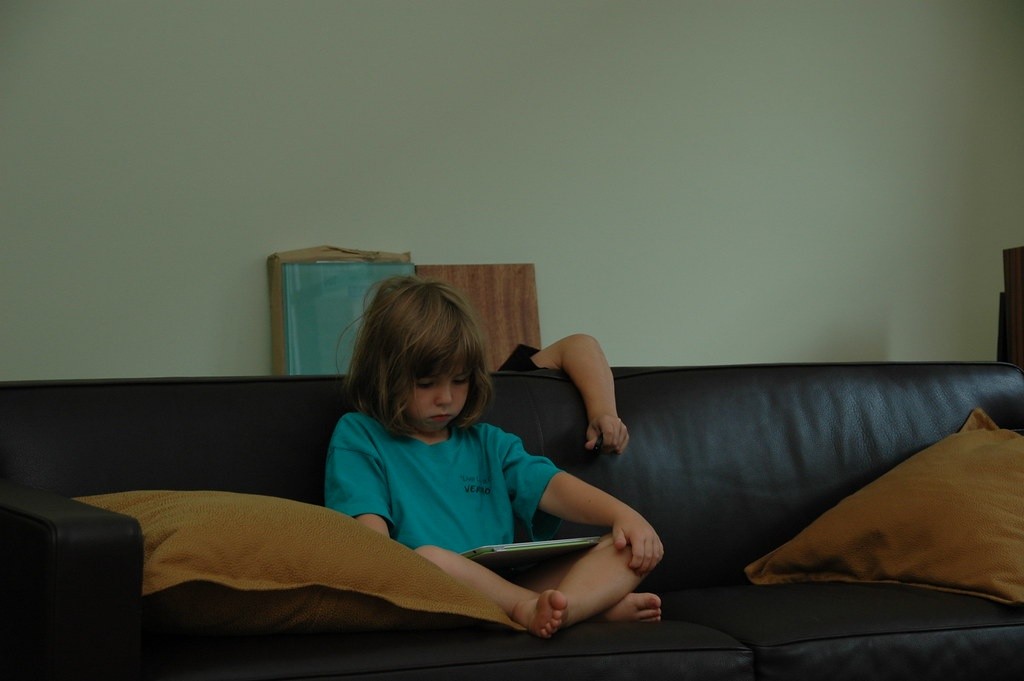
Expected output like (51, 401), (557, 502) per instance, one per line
(68, 489), (530, 632)
(744, 405), (1024, 607)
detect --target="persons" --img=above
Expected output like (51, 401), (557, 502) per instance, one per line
(497, 332), (630, 457)
(324, 275), (665, 640)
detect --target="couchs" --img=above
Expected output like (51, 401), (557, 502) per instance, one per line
(0, 358), (1024, 680)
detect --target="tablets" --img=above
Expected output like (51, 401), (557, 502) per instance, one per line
(460, 537), (599, 571)
